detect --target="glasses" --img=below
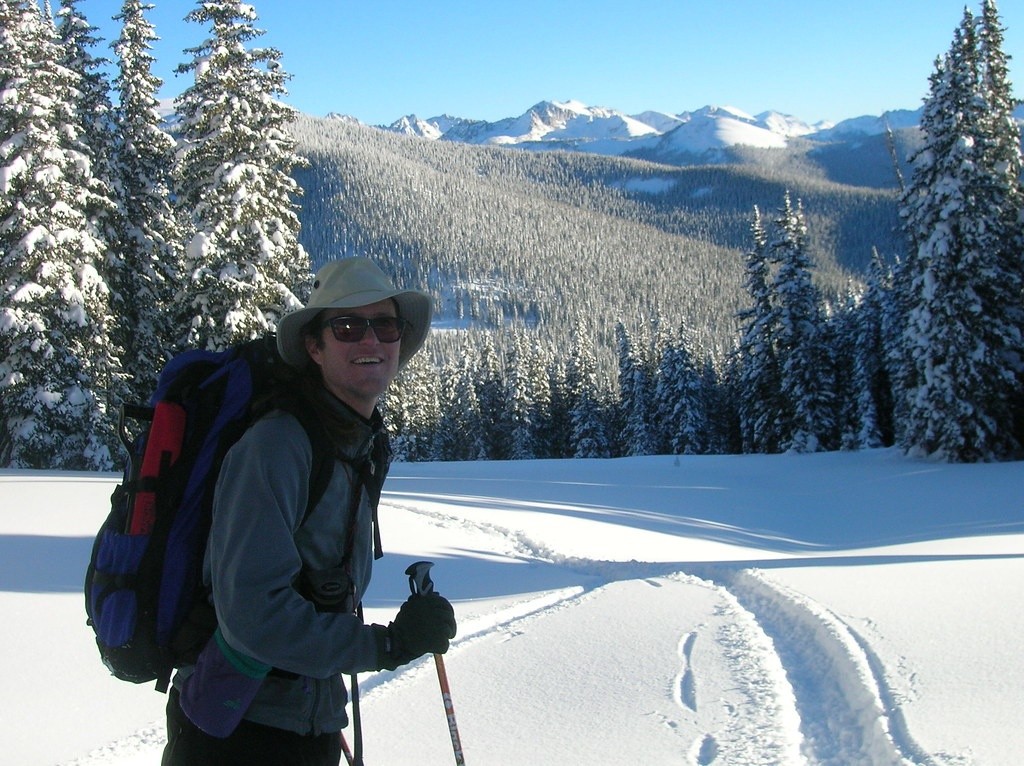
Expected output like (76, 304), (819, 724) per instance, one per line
(321, 314), (404, 343)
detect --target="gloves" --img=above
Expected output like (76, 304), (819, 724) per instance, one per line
(372, 592), (456, 672)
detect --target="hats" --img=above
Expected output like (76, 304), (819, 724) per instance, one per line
(276, 256), (434, 373)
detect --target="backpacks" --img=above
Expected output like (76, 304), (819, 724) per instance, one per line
(84, 342), (336, 694)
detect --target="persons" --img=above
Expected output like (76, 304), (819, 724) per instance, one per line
(161, 255), (457, 765)
